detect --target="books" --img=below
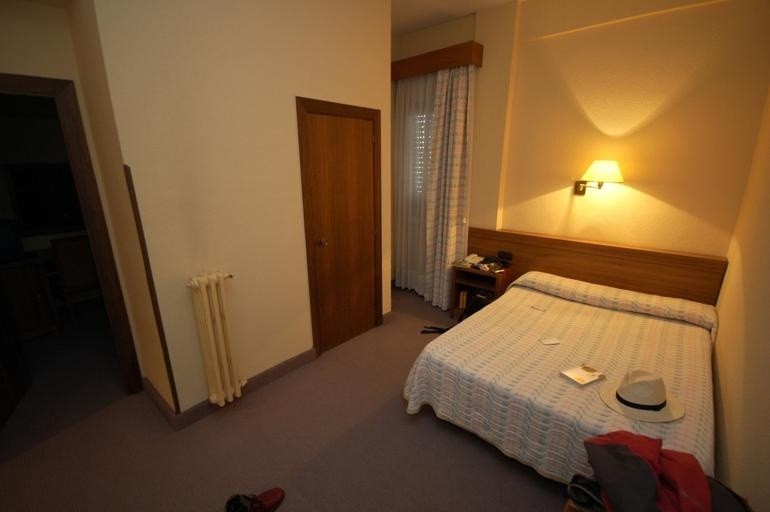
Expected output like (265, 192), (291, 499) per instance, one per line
(561, 362), (606, 388)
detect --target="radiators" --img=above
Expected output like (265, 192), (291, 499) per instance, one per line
(184, 272), (248, 407)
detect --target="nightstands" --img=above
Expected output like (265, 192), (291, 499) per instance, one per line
(448, 260), (514, 321)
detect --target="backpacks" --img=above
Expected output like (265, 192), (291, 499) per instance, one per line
(458, 290), (495, 322)
(566, 429), (714, 512)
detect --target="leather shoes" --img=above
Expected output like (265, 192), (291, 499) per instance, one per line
(223, 487), (285, 512)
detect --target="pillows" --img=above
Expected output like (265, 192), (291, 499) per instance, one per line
(520, 270), (715, 330)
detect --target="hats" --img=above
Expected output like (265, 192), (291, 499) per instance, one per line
(498, 250), (514, 266)
(599, 365), (687, 425)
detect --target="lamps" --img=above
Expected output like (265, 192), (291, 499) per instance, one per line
(573, 159), (625, 196)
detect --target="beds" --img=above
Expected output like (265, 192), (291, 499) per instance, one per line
(421, 285), (716, 488)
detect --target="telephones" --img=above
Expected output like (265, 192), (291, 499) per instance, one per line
(463, 253), (485, 267)
